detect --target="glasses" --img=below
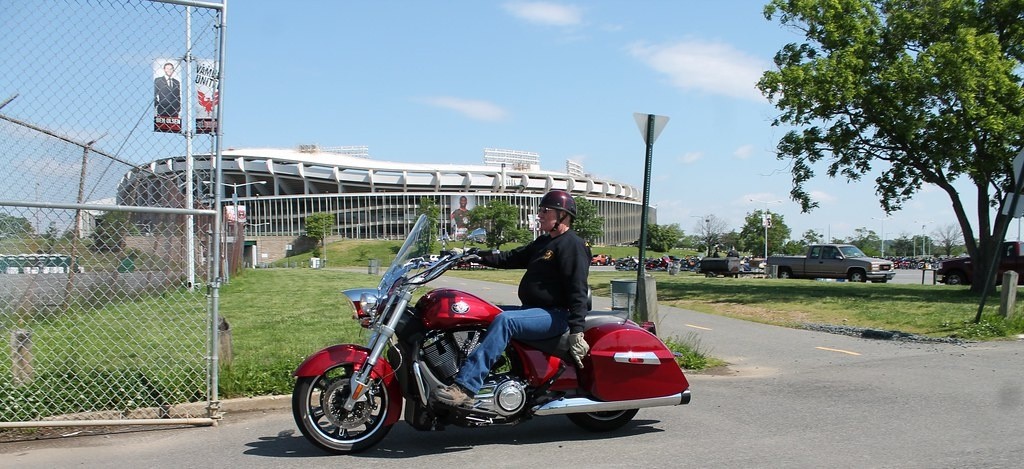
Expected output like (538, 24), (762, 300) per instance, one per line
(538, 208), (556, 214)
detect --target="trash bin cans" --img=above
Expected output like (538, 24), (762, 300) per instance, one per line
(610, 279), (638, 322)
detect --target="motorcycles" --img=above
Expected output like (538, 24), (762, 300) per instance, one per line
(288, 213), (696, 451)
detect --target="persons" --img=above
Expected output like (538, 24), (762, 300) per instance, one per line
(430, 190), (592, 410)
(450, 195), (470, 229)
(153, 62), (181, 117)
(726, 245), (739, 278)
(712, 247), (720, 258)
(605, 253), (654, 266)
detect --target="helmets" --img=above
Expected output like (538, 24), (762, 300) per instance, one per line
(539, 191), (578, 219)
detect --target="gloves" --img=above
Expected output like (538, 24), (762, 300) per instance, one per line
(451, 245), (471, 266)
(568, 330), (589, 369)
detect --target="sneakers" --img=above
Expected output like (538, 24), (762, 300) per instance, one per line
(431, 383), (475, 410)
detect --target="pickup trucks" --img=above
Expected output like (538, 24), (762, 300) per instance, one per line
(769, 243), (896, 284)
(936, 242), (1024, 294)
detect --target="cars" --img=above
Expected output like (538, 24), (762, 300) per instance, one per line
(591, 254), (617, 265)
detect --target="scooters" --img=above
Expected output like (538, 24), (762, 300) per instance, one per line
(616, 254), (768, 274)
(872, 254), (965, 271)
